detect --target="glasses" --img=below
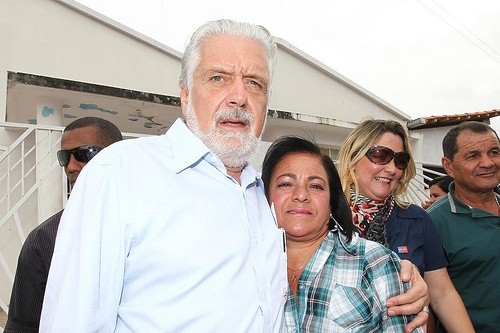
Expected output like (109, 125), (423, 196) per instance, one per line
(56, 144), (102, 167)
(367, 145), (411, 170)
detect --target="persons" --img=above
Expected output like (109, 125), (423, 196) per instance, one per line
(3, 117), (123, 333)
(261, 134), (422, 333)
(335, 117), (475, 333)
(39, 20), (431, 333)
(430, 176), (454, 204)
(422, 121), (500, 333)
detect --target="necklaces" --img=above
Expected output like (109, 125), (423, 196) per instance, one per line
(459, 193), (500, 209)
(288, 265), (304, 283)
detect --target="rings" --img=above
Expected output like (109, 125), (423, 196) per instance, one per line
(422, 306), (430, 314)
